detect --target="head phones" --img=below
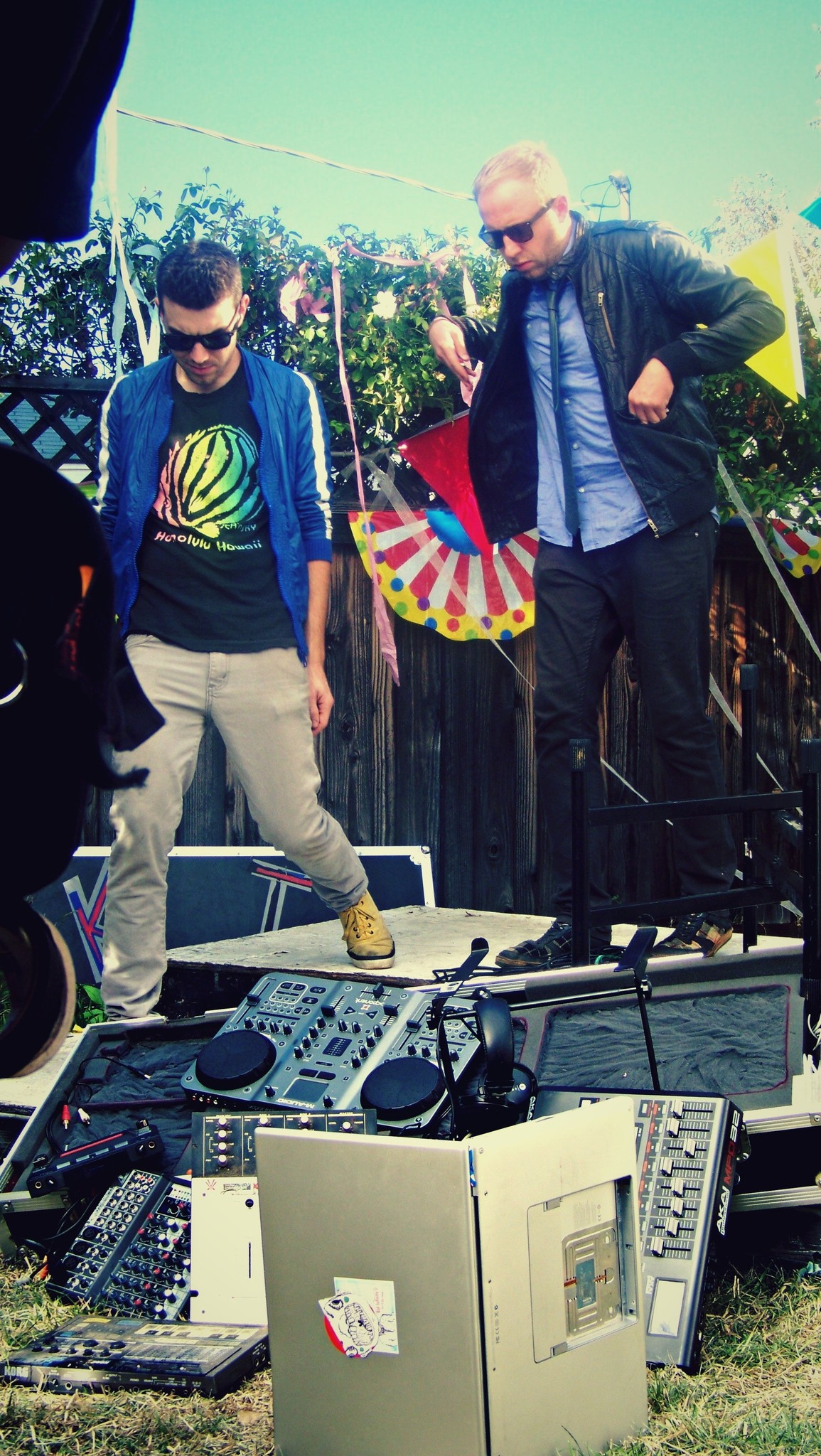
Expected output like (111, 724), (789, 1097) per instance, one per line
(454, 995), (538, 1136)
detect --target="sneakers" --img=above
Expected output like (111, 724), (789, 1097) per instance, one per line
(497, 918), (614, 967)
(332, 889), (394, 969)
(649, 912), (732, 959)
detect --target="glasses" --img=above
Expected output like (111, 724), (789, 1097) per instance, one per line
(158, 306), (242, 351)
(480, 199), (557, 248)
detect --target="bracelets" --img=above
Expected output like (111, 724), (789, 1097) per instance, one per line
(427, 319), (448, 336)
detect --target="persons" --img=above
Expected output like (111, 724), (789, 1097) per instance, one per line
(426, 143), (787, 967)
(95, 241), (395, 1023)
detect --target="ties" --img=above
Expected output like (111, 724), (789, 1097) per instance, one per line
(544, 270), (582, 536)
